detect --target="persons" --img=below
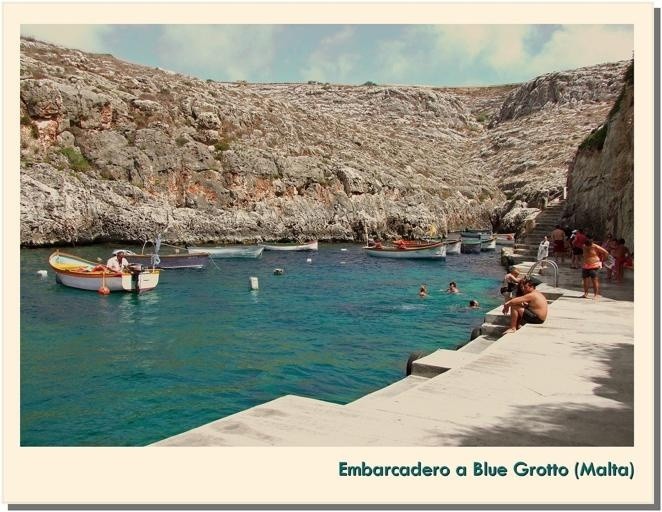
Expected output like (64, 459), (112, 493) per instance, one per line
(537, 235), (550, 274)
(500, 270), (521, 303)
(392, 239), (406, 249)
(447, 281), (459, 293)
(417, 285), (427, 298)
(374, 239), (384, 249)
(468, 300), (480, 309)
(551, 225), (630, 300)
(106, 251), (129, 273)
(502, 280), (548, 334)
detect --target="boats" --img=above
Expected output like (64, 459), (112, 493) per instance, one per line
(112, 250), (210, 270)
(49, 249), (159, 294)
(363, 228), (515, 260)
(186, 245), (263, 259)
(257, 241), (318, 252)
